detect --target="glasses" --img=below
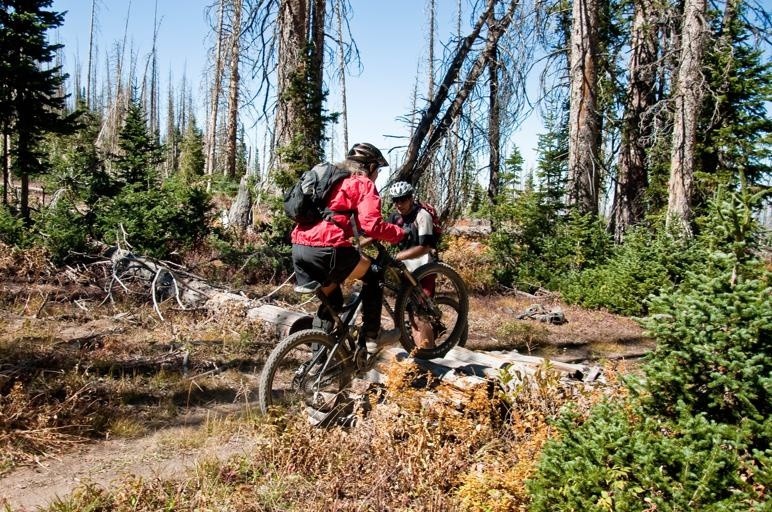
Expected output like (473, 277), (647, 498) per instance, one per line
(395, 196), (408, 204)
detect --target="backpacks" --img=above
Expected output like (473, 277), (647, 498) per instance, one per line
(282, 159), (364, 225)
(411, 201), (442, 244)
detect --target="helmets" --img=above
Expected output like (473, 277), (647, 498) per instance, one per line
(388, 180), (415, 200)
(344, 141), (392, 169)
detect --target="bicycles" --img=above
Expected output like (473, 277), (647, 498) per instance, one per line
(257, 232), (470, 434)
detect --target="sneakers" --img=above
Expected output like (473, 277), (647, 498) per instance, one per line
(366, 325), (402, 355)
(304, 359), (337, 377)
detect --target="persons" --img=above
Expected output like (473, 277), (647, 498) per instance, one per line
(384, 181), (441, 359)
(292, 142), (412, 376)
